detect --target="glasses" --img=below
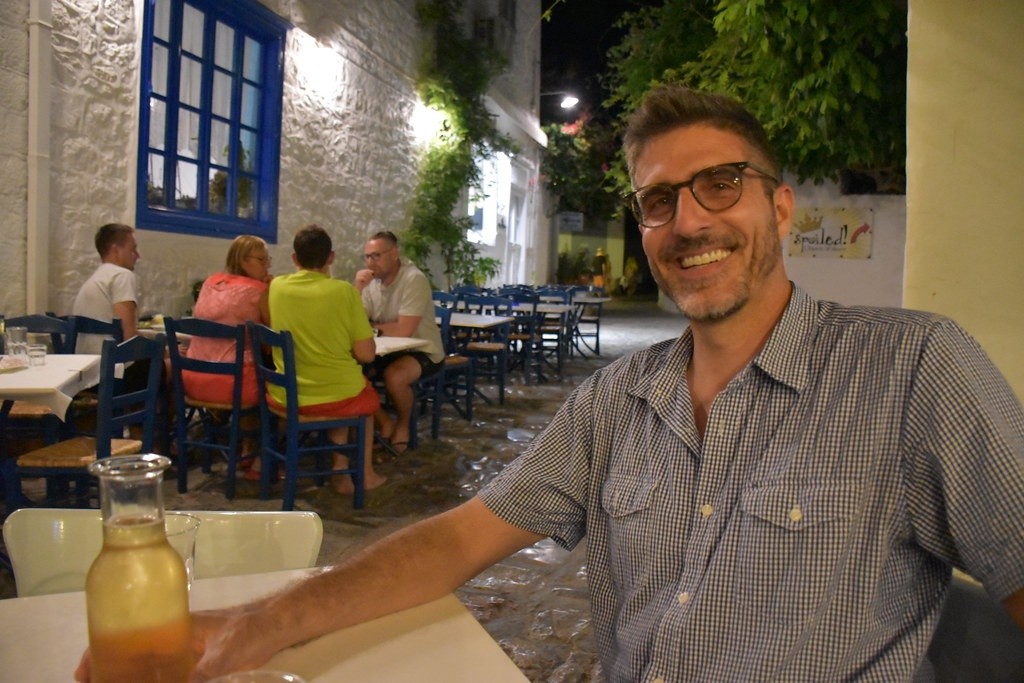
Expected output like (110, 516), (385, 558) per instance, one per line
(623, 162), (781, 227)
(362, 247), (394, 263)
(244, 255), (272, 264)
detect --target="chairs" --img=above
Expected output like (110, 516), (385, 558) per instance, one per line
(0, 281), (604, 507)
(2, 509), (324, 598)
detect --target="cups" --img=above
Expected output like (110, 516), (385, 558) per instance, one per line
(205, 670), (302, 683)
(28, 344), (47, 366)
(373, 328), (378, 337)
(6, 342), (28, 361)
(163, 512), (201, 593)
(6, 326), (27, 344)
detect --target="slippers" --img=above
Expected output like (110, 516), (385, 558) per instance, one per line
(372, 436), (411, 464)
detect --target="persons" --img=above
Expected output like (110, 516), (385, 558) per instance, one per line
(591, 247), (611, 298)
(73, 222), (189, 456)
(267, 223), (388, 495)
(182, 235), (286, 483)
(354, 232), (446, 454)
(624, 256), (638, 298)
(73, 87), (1024, 683)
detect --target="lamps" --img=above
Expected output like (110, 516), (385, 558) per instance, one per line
(540, 91), (579, 108)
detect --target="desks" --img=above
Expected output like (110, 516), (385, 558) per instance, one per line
(0, 354), (123, 524)
(527, 295), (613, 361)
(0, 564), (530, 683)
(435, 312), (515, 419)
(137, 323), (439, 458)
(433, 299), (579, 380)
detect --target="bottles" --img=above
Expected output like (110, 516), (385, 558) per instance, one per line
(88, 453), (190, 683)
(0, 315), (4, 355)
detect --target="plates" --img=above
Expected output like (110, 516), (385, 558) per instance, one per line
(150, 324), (165, 330)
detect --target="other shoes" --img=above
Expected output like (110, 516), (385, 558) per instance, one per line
(246, 469), (284, 482)
(237, 460), (249, 470)
